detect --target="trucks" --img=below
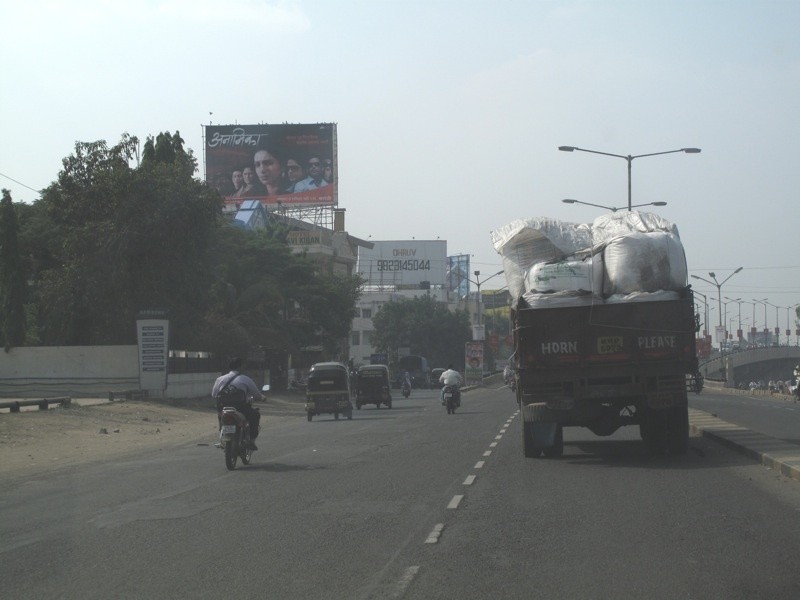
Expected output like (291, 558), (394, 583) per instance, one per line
(398, 355), (427, 389)
(510, 291), (694, 460)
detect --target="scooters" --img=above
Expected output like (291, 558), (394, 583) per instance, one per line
(504, 375), (517, 393)
(401, 380), (410, 398)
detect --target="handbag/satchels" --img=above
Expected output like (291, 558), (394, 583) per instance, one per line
(217, 384), (247, 413)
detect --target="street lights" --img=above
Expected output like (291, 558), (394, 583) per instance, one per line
(453, 270), (504, 325)
(705, 295), (800, 365)
(557, 145), (701, 213)
(691, 266), (743, 381)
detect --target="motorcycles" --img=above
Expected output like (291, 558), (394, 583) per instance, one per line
(356, 364), (392, 410)
(214, 384), (271, 471)
(430, 368), (446, 390)
(438, 382), (460, 415)
(305, 362), (353, 422)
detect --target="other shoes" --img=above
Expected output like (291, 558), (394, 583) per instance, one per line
(249, 441), (257, 450)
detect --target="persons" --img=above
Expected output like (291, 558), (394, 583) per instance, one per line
(439, 363), (463, 406)
(402, 370), (411, 388)
(212, 359), (267, 451)
(748, 378), (793, 394)
(503, 365), (516, 383)
(230, 140), (332, 197)
(470, 357), (479, 368)
